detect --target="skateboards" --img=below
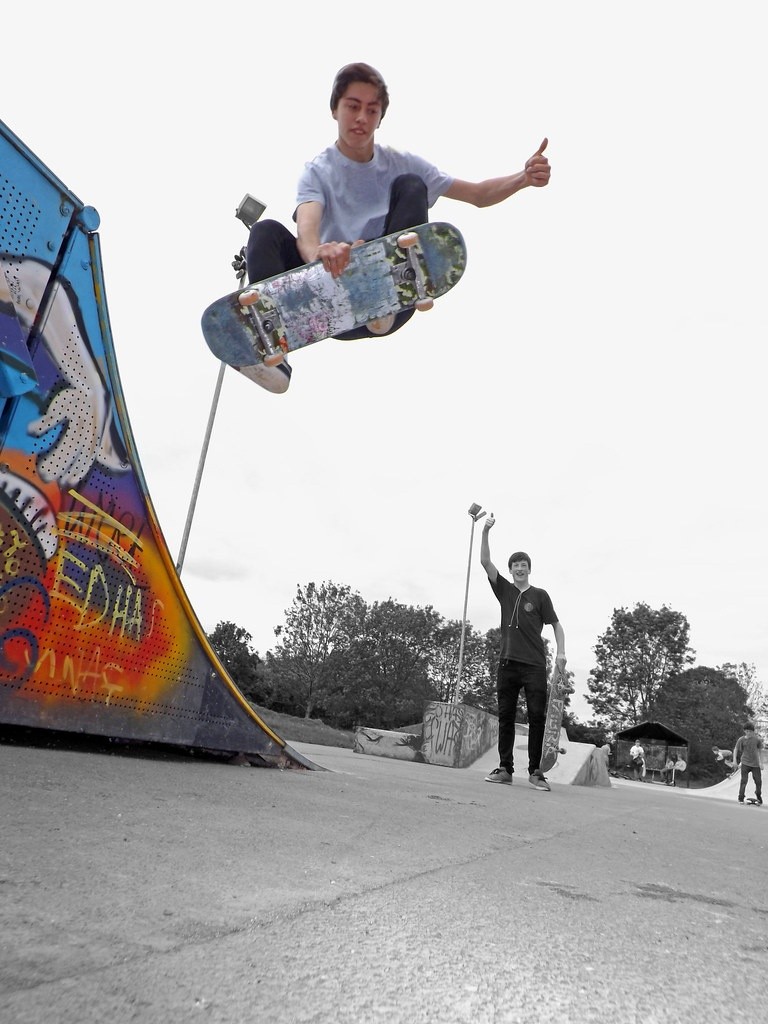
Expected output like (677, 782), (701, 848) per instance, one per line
(747, 797), (761, 805)
(200, 221), (467, 369)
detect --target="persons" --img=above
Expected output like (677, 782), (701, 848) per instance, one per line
(601, 742), (612, 771)
(630, 740), (645, 782)
(480, 513), (567, 791)
(660, 754), (674, 783)
(666, 753), (686, 786)
(712, 746), (739, 771)
(227, 63), (551, 394)
(731, 723), (764, 804)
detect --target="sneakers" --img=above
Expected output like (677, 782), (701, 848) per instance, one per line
(756, 794), (764, 805)
(363, 312), (399, 335)
(223, 346), (295, 396)
(485, 766), (513, 785)
(527, 769), (551, 793)
(737, 795), (746, 803)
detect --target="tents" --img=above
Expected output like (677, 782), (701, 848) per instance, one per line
(612, 721), (690, 788)
